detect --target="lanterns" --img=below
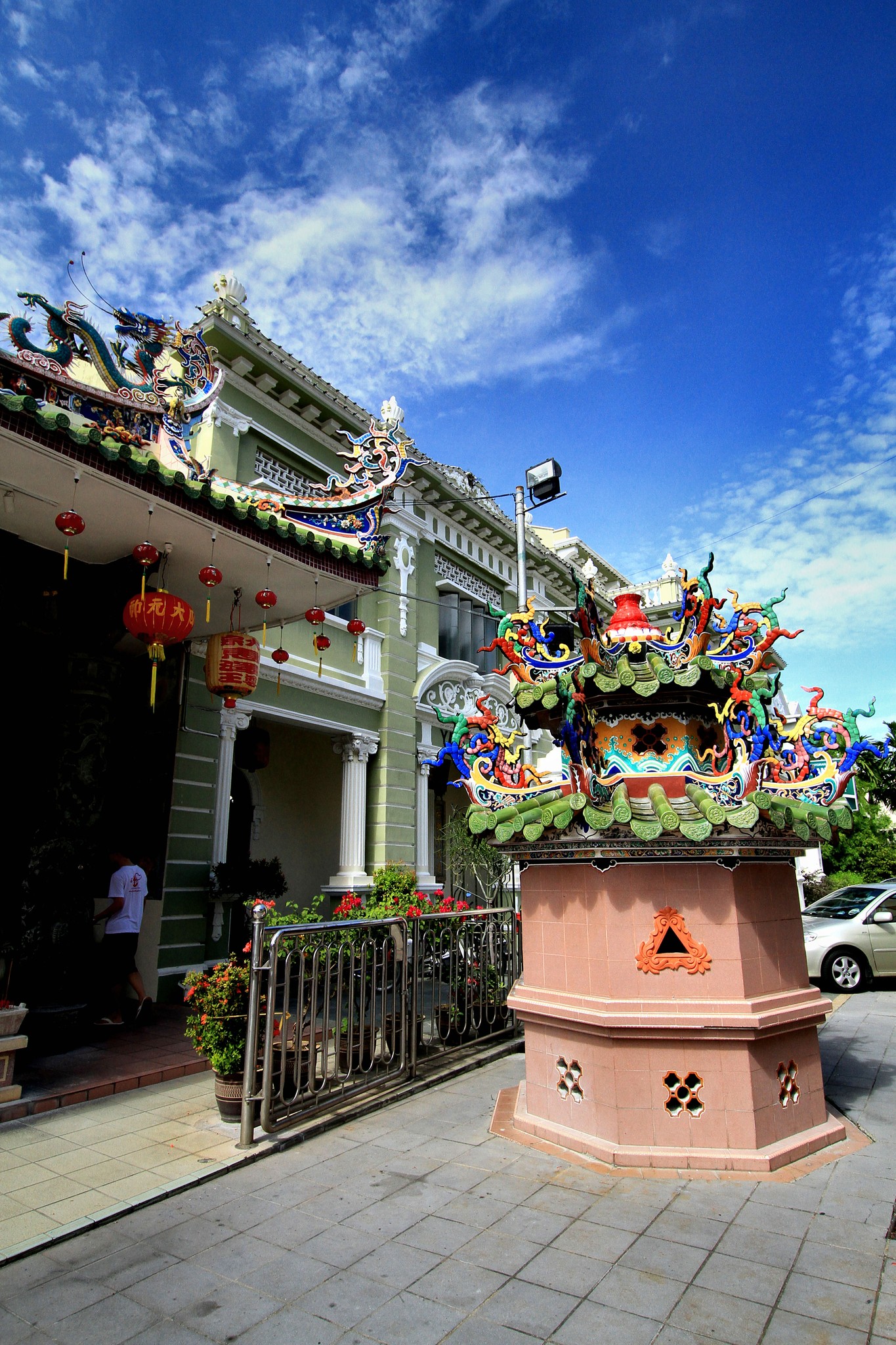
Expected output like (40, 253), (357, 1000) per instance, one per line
(56, 487), (366, 711)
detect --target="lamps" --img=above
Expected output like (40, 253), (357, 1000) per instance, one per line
(525, 456), (562, 506)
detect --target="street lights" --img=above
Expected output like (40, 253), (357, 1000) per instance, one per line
(514, 455), (563, 768)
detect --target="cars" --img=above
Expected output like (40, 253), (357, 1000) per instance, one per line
(798, 877), (895, 992)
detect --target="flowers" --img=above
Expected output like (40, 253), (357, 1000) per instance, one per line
(183, 947), (276, 1078)
(368, 862), (435, 1021)
(447, 942), (507, 1006)
(246, 904), (356, 1046)
(331, 889), (396, 1027)
(416, 888), (490, 1026)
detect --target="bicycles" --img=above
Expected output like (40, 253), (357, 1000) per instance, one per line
(352, 909), (509, 992)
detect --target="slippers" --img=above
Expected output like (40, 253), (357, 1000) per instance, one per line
(94, 1017), (124, 1025)
(136, 996), (152, 1025)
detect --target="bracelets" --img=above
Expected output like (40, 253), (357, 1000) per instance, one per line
(92, 916), (96, 925)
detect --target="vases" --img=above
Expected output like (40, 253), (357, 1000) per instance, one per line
(329, 1020), (377, 1073)
(214, 1056), (273, 1126)
(270, 1036), (322, 1096)
(472, 996), (510, 1032)
(384, 1009), (427, 1054)
(435, 1002), (473, 1041)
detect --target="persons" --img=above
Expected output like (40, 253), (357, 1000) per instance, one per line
(87, 843), (155, 1028)
